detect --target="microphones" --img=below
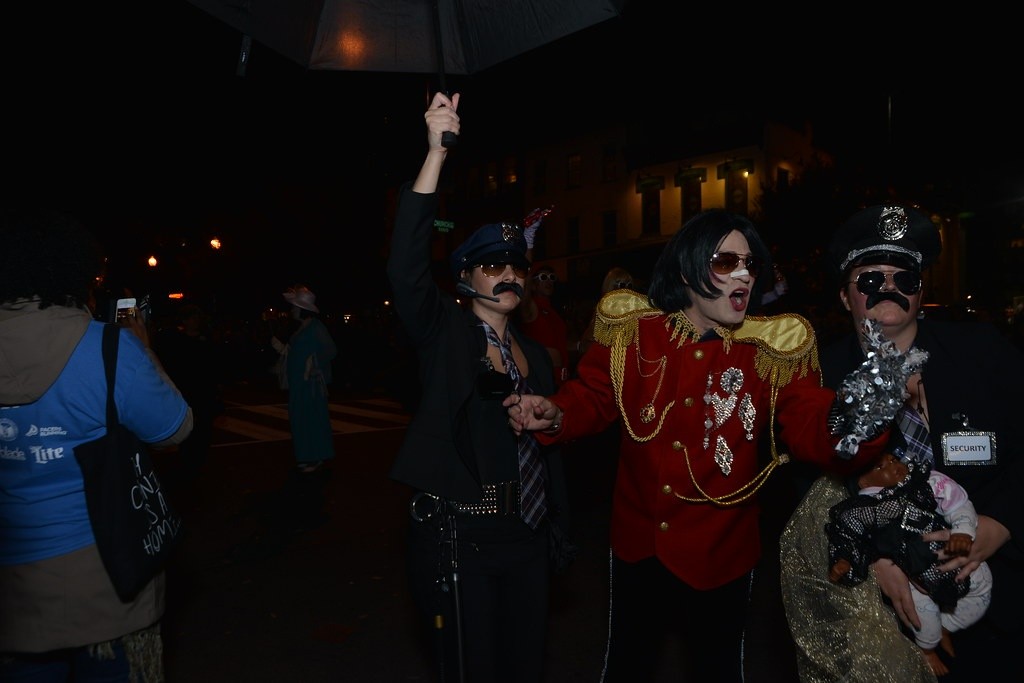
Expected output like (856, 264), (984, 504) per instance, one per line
(456, 283), (499, 302)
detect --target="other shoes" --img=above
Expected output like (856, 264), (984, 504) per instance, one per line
(296, 461), (323, 473)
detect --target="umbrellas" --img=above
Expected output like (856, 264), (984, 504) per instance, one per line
(189, 1), (618, 149)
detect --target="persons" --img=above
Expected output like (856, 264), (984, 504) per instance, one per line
(501, 206), (931, 683)
(0, 232), (226, 683)
(824, 431), (994, 677)
(269, 282), (339, 516)
(779, 197), (1024, 683)
(389, 90), (571, 683)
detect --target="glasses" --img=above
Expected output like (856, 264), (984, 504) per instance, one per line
(474, 261), (529, 279)
(290, 306), (300, 311)
(709, 252), (765, 278)
(536, 272), (558, 281)
(851, 270), (923, 296)
(617, 282), (634, 289)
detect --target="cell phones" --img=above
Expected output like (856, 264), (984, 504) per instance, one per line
(114, 297), (137, 323)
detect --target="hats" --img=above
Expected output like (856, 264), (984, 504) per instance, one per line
(284, 285), (320, 314)
(833, 202), (942, 281)
(460, 221), (532, 268)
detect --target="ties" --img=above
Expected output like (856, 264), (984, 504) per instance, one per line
(483, 322), (548, 531)
(895, 401), (935, 470)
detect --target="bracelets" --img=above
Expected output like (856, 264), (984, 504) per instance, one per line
(542, 407), (562, 434)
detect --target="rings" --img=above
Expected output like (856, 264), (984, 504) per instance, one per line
(509, 390), (523, 401)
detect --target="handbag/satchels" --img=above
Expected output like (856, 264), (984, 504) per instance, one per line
(74, 322), (187, 604)
(268, 343), (288, 389)
